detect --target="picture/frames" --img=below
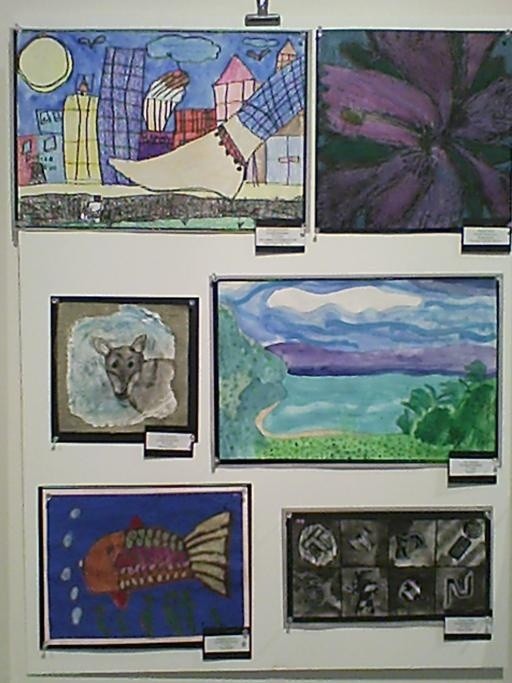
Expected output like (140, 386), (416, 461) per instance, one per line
(36, 480), (258, 648)
(205, 268), (506, 474)
(278, 500), (499, 630)
(48, 293), (201, 449)
